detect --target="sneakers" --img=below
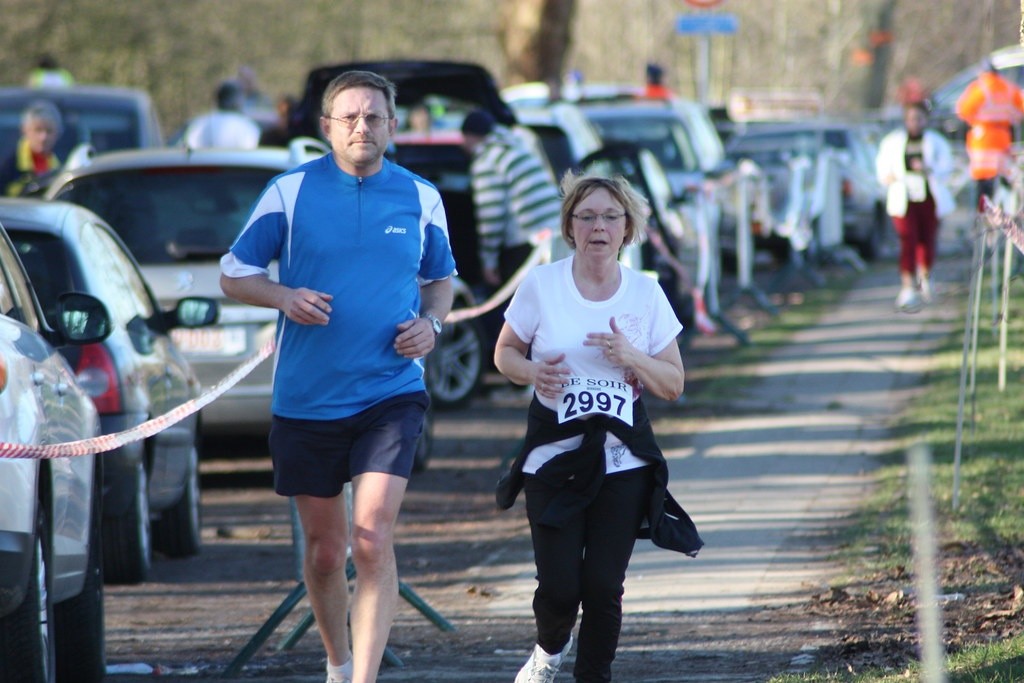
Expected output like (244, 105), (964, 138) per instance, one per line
(514, 631), (574, 683)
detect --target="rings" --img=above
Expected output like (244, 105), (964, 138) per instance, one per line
(609, 348), (612, 354)
(541, 383), (545, 390)
(608, 340), (611, 347)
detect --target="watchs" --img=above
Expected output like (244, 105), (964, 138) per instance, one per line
(422, 312), (442, 337)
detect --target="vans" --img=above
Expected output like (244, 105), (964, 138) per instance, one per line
(0, 83), (164, 202)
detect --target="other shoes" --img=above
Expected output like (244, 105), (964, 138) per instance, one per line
(895, 289), (914, 307)
(920, 281), (933, 299)
(491, 383), (537, 408)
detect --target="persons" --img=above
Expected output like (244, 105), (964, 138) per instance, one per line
(220, 71), (458, 683)
(876, 103), (955, 308)
(493, 177), (705, 683)
(0, 101), (62, 197)
(638, 63), (672, 98)
(955, 62), (1023, 215)
(187, 72), (295, 147)
(29, 53), (72, 87)
(406, 103), (560, 407)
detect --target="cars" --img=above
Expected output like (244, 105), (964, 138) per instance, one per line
(282, 60), (906, 374)
(0, 194), (221, 587)
(44, 134), (486, 474)
(0, 217), (114, 683)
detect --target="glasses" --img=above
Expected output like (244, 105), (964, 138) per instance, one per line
(325, 111), (392, 128)
(569, 208), (628, 224)
